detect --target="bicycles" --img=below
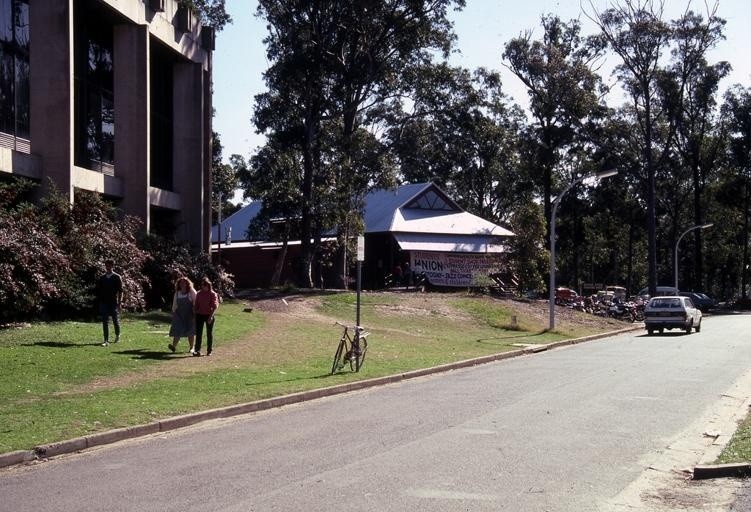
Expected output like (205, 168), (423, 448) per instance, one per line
(331, 322), (371, 375)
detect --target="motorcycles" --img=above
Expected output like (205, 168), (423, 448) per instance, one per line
(571, 293), (650, 324)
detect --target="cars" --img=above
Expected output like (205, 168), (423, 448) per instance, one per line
(643, 296), (702, 336)
(669, 292), (713, 312)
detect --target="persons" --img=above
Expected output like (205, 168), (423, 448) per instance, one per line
(94, 260), (123, 347)
(395, 259), (422, 287)
(168, 277), (219, 356)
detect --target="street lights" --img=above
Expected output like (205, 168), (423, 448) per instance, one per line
(674, 223), (713, 295)
(549, 168), (618, 328)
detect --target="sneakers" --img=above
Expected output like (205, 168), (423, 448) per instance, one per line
(190, 347), (211, 356)
(169, 344), (176, 351)
(102, 335), (120, 346)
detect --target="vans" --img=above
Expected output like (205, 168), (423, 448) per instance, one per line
(637, 286), (675, 297)
